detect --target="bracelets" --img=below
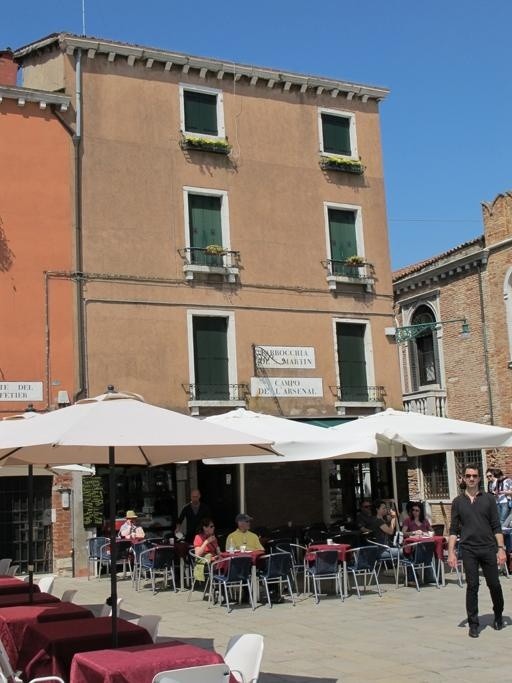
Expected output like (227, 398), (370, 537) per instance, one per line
(498, 545), (506, 550)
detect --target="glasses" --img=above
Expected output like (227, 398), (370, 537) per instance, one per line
(466, 474), (477, 478)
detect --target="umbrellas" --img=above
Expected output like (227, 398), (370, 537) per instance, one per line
(0, 385), (284, 645)
(148, 407), (379, 515)
(0, 405), (99, 603)
(328, 407), (512, 516)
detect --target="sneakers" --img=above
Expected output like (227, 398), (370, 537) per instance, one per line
(408, 581), (417, 587)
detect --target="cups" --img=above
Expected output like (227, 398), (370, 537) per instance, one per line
(326, 538), (333, 545)
(239, 545), (246, 553)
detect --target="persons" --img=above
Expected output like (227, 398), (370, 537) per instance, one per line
(225, 515), (265, 551)
(193, 518), (220, 557)
(120, 510), (145, 539)
(484, 467), (512, 525)
(357, 500), (431, 560)
(447, 464), (507, 636)
(176, 489), (209, 546)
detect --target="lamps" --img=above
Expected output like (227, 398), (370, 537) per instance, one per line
(394, 315), (471, 345)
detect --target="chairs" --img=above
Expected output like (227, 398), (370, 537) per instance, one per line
(89, 536), (187, 595)
(0, 555), (266, 683)
(188, 522), (464, 613)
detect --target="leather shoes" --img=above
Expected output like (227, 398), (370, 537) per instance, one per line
(469, 628), (478, 638)
(494, 620), (502, 630)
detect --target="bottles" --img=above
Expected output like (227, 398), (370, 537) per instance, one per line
(229, 538), (234, 555)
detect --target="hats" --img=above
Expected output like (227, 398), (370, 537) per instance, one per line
(235, 514), (253, 523)
(125, 510), (138, 518)
